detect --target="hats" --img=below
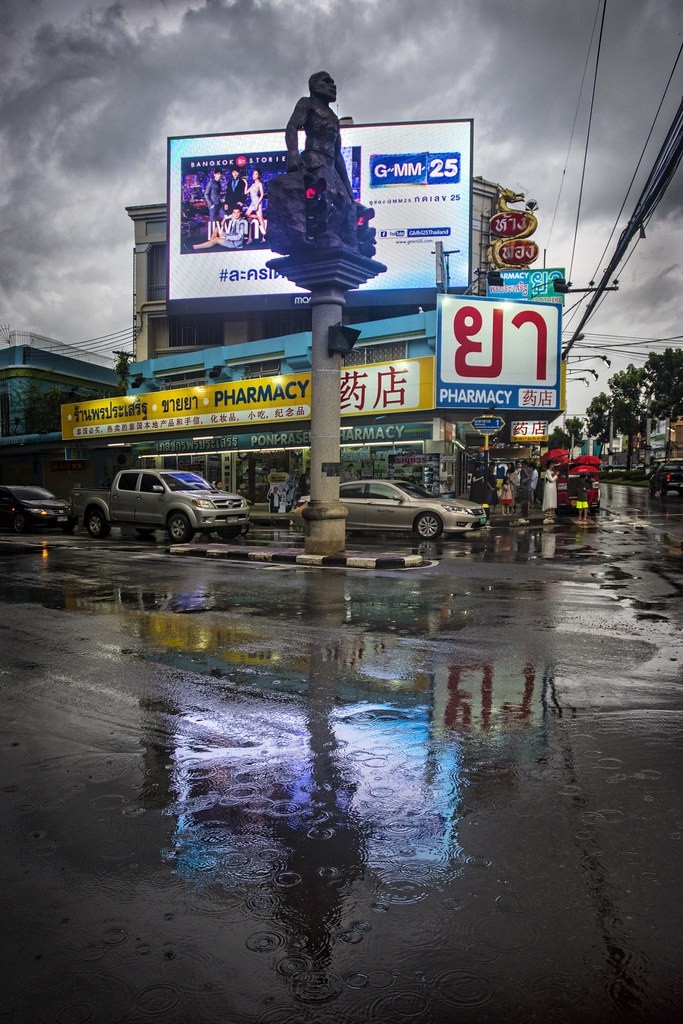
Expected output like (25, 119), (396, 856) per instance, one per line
(489, 462), (498, 466)
(474, 462), (482, 467)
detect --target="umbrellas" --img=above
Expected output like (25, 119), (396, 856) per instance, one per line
(574, 455), (601, 465)
(568, 464), (600, 474)
(554, 456), (569, 464)
(541, 448), (570, 472)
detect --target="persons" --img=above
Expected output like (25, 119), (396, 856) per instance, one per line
(191, 167), (268, 250)
(285, 70), (360, 250)
(298, 468), (311, 497)
(215, 481), (223, 491)
(470, 459), (600, 524)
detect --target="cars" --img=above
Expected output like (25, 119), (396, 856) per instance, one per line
(0, 484), (74, 535)
(294, 479), (488, 541)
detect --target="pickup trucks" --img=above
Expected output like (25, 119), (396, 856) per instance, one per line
(71, 469), (250, 544)
(648, 462), (683, 497)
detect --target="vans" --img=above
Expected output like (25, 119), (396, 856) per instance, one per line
(554, 462), (600, 515)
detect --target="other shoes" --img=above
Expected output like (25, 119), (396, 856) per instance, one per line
(546, 511), (555, 515)
(501, 513), (507, 516)
